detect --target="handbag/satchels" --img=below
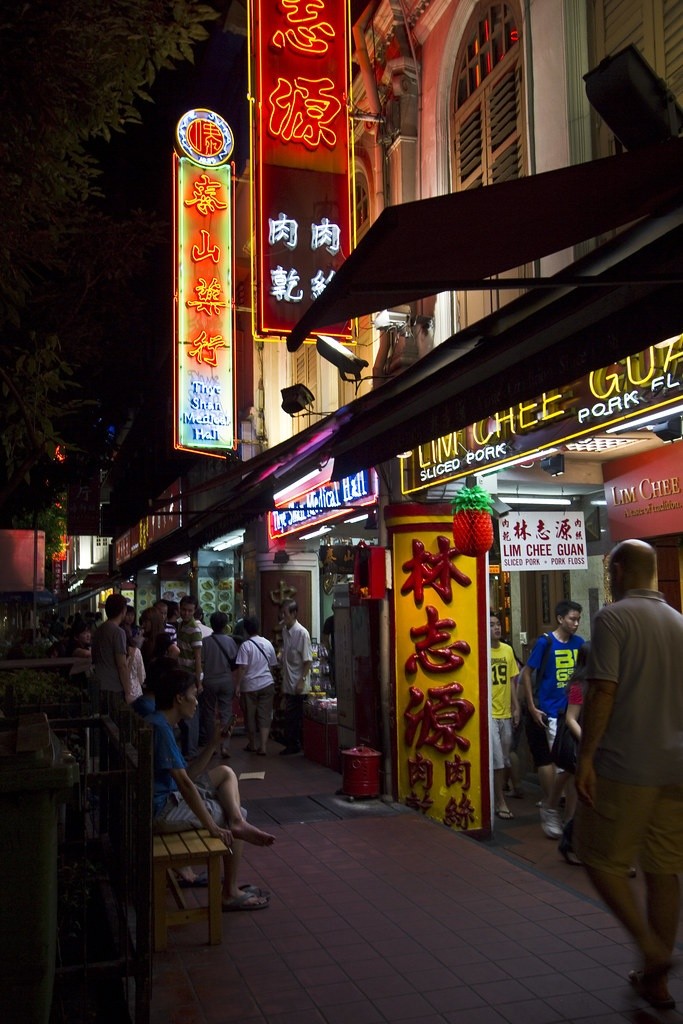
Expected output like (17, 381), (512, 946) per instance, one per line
(228, 657), (240, 672)
(516, 633), (552, 716)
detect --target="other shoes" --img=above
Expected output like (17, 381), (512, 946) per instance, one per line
(558, 844), (584, 865)
(510, 788), (525, 799)
(278, 746), (302, 756)
(243, 743), (256, 752)
(256, 746), (266, 756)
(220, 746), (231, 758)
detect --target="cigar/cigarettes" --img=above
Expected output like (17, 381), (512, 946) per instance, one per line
(279, 620), (284, 624)
(229, 847), (233, 853)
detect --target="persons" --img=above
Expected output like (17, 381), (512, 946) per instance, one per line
(502, 600), (585, 838)
(235, 617), (278, 755)
(41, 611), (102, 650)
(65, 620), (92, 689)
(94, 594), (130, 704)
(119, 599), (180, 705)
(131, 657), (277, 912)
(490, 610), (520, 820)
(558, 641), (637, 877)
(194, 607), (239, 759)
(572, 539), (683, 1009)
(176, 596), (203, 761)
(323, 604), (336, 688)
(278, 599), (313, 755)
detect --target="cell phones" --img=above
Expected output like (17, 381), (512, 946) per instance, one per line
(542, 716), (550, 726)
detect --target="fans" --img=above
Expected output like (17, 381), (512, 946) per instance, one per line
(207, 561), (233, 580)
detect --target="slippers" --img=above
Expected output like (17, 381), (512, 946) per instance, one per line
(495, 809), (515, 820)
(629, 969), (676, 1010)
(177, 871), (225, 889)
(222, 884), (273, 913)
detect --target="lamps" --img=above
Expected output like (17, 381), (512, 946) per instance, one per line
(582, 43), (683, 153)
(273, 550), (290, 564)
(364, 513), (379, 529)
(315, 334), (396, 396)
(280, 384), (334, 418)
(541, 453), (565, 477)
(652, 415), (682, 440)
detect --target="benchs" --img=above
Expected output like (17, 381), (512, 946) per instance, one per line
(153, 826), (229, 951)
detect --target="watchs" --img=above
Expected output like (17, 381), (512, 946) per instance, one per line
(301, 677), (306, 681)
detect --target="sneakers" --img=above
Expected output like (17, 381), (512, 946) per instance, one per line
(539, 807), (563, 839)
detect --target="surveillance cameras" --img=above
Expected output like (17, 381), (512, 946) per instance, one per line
(316, 335), (369, 374)
(487, 495), (512, 520)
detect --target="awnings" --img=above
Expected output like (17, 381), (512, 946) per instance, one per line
(286, 137), (683, 352)
(146, 417), (383, 515)
